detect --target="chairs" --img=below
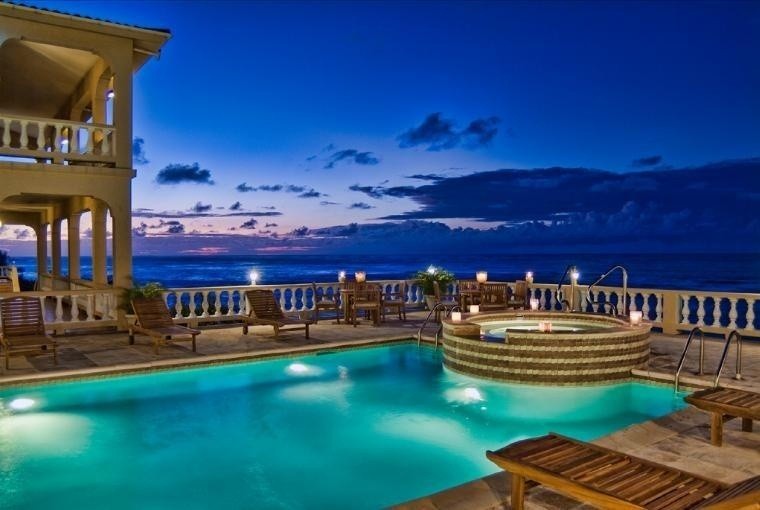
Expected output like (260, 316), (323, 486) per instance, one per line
(486, 385), (760, 510)
(308, 269), (526, 329)
(235, 289), (319, 340)
(2, 295), (63, 371)
(123, 295), (204, 357)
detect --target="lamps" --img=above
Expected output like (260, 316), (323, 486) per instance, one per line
(243, 267), (263, 286)
